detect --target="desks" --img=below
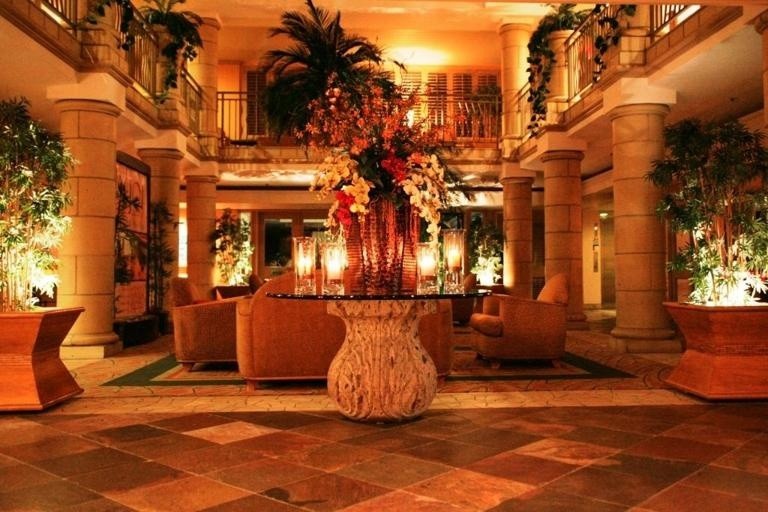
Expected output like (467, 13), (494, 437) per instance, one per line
(267, 285), (491, 421)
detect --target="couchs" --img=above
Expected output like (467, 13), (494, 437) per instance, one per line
(470, 272), (569, 367)
(236, 271), (455, 390)
(451, 273), (476, 323)
(249, 272), (265, 295)
(170, 277), (252, 369)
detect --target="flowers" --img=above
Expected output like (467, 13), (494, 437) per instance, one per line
(299, 83), (473, 231)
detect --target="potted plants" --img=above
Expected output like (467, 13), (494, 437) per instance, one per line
(114, 185), (159, 346)
(648, 118), (768, 402)
(146, 205), (170, 336)
(526, 3), (590, 130)
(128, 0), (203, 102)
(0, 98), (87, 410)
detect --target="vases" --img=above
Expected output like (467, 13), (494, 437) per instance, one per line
(360, 196), (419, 288)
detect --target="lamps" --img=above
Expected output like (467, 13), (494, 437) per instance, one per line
(321, 236), (345, 291)
(417, 244), (438, 288)
(292, 237), (316, 288)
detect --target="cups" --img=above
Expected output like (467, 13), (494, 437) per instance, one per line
(320, 234), (345, 296)
(415, 242), (440, 295)
(293, 237), (316, 296)
